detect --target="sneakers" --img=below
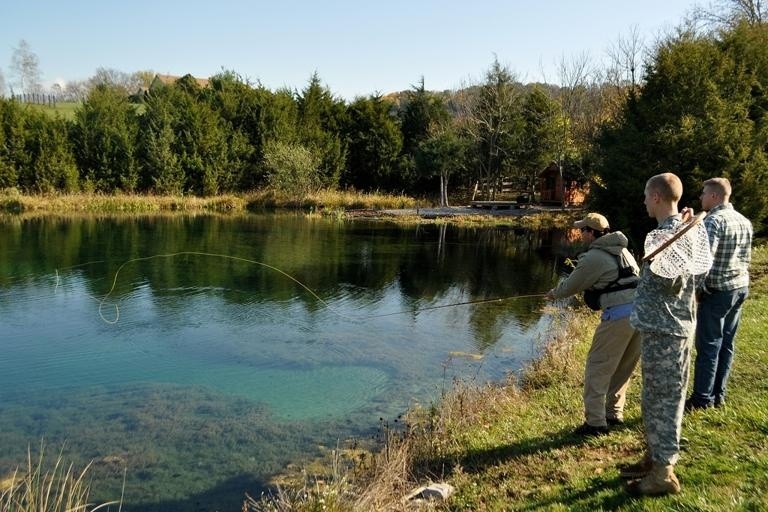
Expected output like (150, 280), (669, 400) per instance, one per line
(685, 397), (726, 411)
(606, 416), (623, 425)
(622, 472), (681, 497)
(574, 422), (607, 435)
(620, 452), (652, 478)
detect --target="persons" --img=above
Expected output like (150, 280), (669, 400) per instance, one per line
(617, 172), (705, 498)
(685, 177), (752, 411)
(546, 212), (641, 436)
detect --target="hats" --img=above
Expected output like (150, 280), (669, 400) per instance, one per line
(573, 212), (610, 233)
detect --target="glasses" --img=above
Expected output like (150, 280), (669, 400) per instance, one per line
(581, 228), (589, 232)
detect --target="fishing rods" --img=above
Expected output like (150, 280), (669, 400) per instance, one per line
(358, 293), (549, 321)
